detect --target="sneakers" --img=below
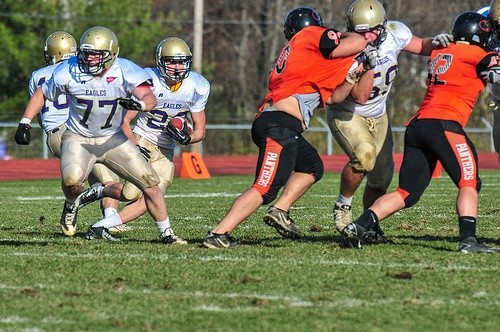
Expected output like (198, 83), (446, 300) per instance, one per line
(78, 183), (102, 210)
(162, 235), (187, 245)
(202, 231), (241, 251)
(264, 205), (304, 239)
(85, 225), (120, 242)
(363, 221), (394, 244)
(61, 198), (79, 236)
(459, 236), (499, 253)
(332, 201), (352, 233)
(108, 224), (134, 232)
(343, 222), (368, 248)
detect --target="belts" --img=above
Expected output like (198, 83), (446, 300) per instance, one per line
(46, 128), (59, 134)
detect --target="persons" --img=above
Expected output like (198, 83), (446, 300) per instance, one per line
(28, 30), (131, 232)
(342, 0), (500, 253)
(203, 8), (368, 249)
(328, 0), (454, 246)
(78, 36), (210, 240)
(14, 27), (188, 245)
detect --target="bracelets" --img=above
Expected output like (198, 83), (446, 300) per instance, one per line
(137, 100), (146, 111)
(20, 118), (31, 124)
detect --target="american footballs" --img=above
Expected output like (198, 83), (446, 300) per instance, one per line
(170, 116), (193, 135)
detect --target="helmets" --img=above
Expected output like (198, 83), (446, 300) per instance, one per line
(156, 38), (192, 85)
(44, 31), (78, 65)
(477, 6), (490, 18)
(284, 8), (323, 40)
(347, 0), (387, 32)
(489, 0), (500, 26)
(452, 12), (491, 48)
(77, 27), (121, 75)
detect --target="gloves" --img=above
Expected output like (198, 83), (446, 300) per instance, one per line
(166, 122), (191, 146)
(363, 45), (378, 71)
(119, 98), (142, 111)
(15, 123), (30, 145)
(480, 69), (500, 84)
(431, 33), (454, 47)
